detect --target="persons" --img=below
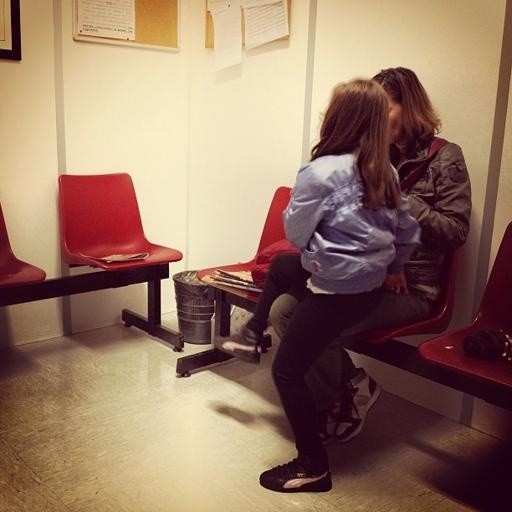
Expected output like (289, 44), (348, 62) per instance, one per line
(257, 66), (473, 493)
(213, 77), (423, 444)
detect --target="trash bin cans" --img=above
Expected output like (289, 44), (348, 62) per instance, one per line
(173, 271), (215, 344)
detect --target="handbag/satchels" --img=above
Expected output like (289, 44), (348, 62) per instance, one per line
(251, 238), (302, 289)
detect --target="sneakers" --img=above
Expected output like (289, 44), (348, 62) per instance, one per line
(334, 367), (384, 444)
(214, 325), (265, 365)
(318, 389), (344, 445)
(260, 458), (332, 494)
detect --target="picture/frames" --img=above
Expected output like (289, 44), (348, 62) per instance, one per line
(0, 0), (22, 61)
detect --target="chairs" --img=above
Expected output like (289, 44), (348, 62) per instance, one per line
(57, 171), (183, 272)
(0, 202), (46, 288)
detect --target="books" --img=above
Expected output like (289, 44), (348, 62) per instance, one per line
(210, 269), (265, 294)
